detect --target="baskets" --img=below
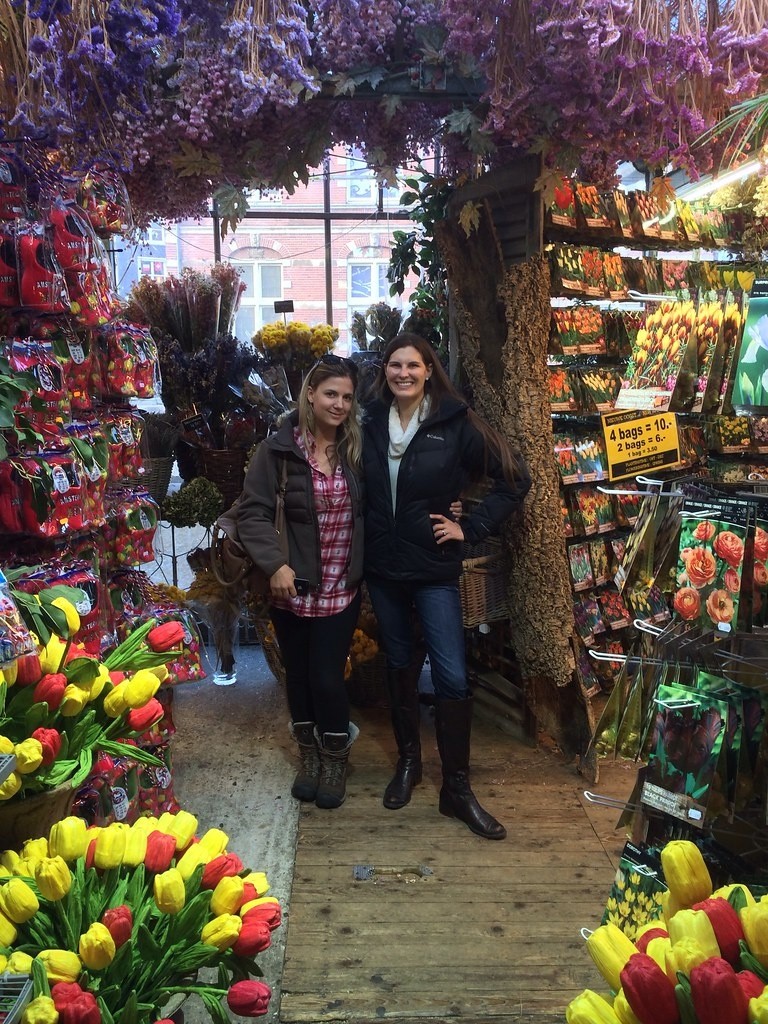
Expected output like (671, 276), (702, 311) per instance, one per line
(0, 776), (84, 854)
(174, 441), (250, 494)
(110, 456), (175, 506)
(458, 513), (511, 629)
(458, 472), (493, 509)
(247, 602), (286, 687)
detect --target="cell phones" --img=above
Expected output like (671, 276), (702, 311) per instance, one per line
(293, 578), (310, 596)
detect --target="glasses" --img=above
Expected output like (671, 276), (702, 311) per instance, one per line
(309, 354), (358, 384)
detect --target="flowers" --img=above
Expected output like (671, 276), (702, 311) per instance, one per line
(563, 841), (768, 1024)
(123, 261), (254, 410)
(253, 321), (338, 365)
(0, 809), (281, 1024)
(0, 589), (187, 799)
(0, 1), (768, 190)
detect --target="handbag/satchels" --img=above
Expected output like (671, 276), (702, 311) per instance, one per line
(211, 453), (289, 623)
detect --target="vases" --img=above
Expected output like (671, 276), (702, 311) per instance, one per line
(287, 368), (304, 401)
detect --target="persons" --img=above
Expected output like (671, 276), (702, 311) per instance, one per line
(235, 355), (464, 809)
(358, 333), (531, 839)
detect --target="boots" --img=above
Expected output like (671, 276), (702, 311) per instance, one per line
(382, 692), (423, 811)
(288, 721), (320, 802)
(313, 720), (360, 810)
(436, 692), (507, 840)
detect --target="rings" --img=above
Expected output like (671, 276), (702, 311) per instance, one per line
(441, 529), (446, 536)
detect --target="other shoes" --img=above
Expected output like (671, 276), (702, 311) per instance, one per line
(0, 143), (207, 829)
